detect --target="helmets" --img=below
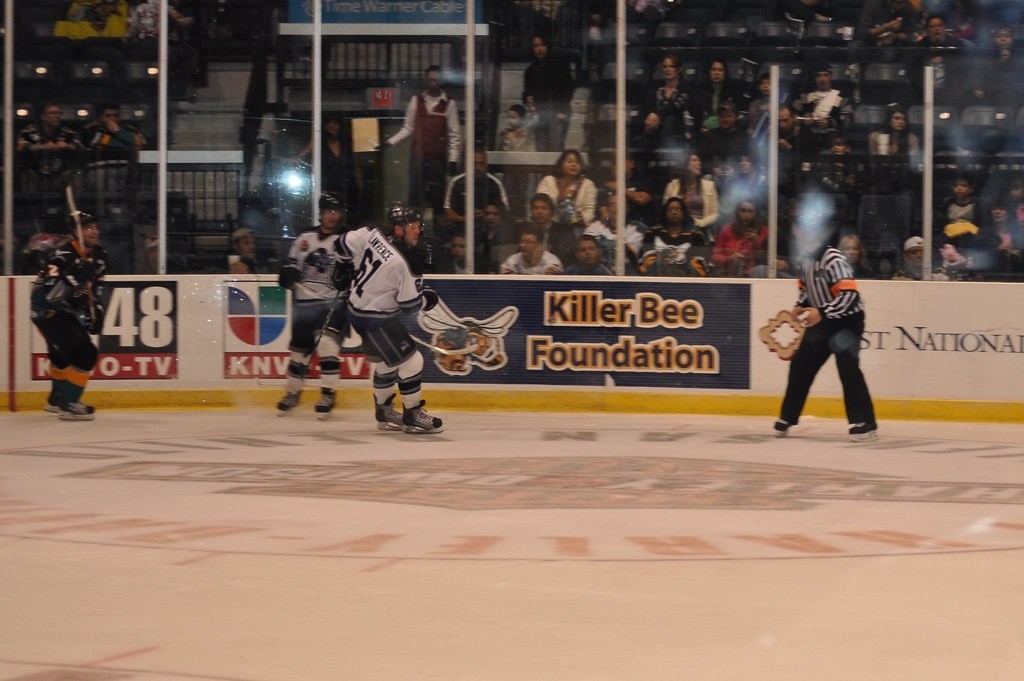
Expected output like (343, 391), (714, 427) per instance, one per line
(70, 213), (98, 229)
(319, 193), (345, 210)
(390, 207), (421, 222)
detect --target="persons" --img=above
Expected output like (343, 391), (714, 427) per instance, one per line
(29, 212), (105, 421)
(275, 195), (347, 420)
(229, 228), (267, 274)
(333, 207), (445, 434)
(774, 215), (878, 441)
(272, 0), (1024, 282)
(16, 0), (193, 275)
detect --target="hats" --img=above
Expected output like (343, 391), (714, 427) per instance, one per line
(716, 101), (737, 114)
(904, 236), (923, 251)
(231, 227), (256, 242)
(144, 236), (157, 255)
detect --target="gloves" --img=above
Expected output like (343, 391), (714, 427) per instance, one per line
(280, 268), (299, 289)
(88, 303), (105, 335)
(332, 263), (354, 292)
(422, 287), (438, 311)
(72, 260), (92, 283)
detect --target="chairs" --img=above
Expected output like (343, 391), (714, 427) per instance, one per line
(0, 0), (1023, 281)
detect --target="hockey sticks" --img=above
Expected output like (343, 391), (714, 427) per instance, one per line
(65, 184), (96, 322)
(295, 282), (480, 357)
(303, 290), (341, 366)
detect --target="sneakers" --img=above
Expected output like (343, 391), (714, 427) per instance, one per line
(46, 391), (69, 412)
(373, 394), (404, 430)
(315, 391), (337, 420)
(401, 399), (443, 434)
(849, 422), (878, 443)
(775, 419), (791, 437)
(275, 390), (302, 415)
(58, 398), (95, 421)
